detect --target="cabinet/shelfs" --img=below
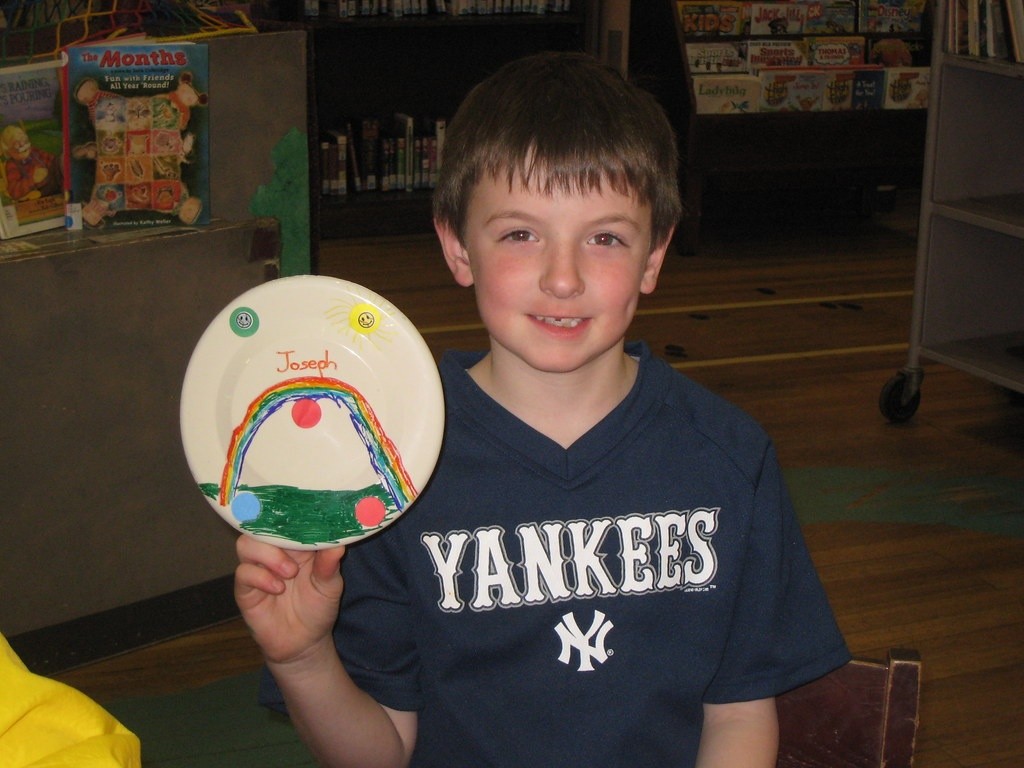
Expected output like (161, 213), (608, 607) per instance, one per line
(878, 0), (1024, 425)
(248, 0), (600, 240)
(629, 0), (939, 257)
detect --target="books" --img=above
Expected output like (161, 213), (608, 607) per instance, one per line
(943, 1), (1024, 63)
(321, 111), (446, 195)
(1, 33), (211, 240)
(305, 0), (571, 17)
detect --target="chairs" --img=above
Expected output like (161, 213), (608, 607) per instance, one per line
(773, 642), (924, 768)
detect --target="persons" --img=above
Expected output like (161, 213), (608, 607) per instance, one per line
(232, 51), (850, 767)
